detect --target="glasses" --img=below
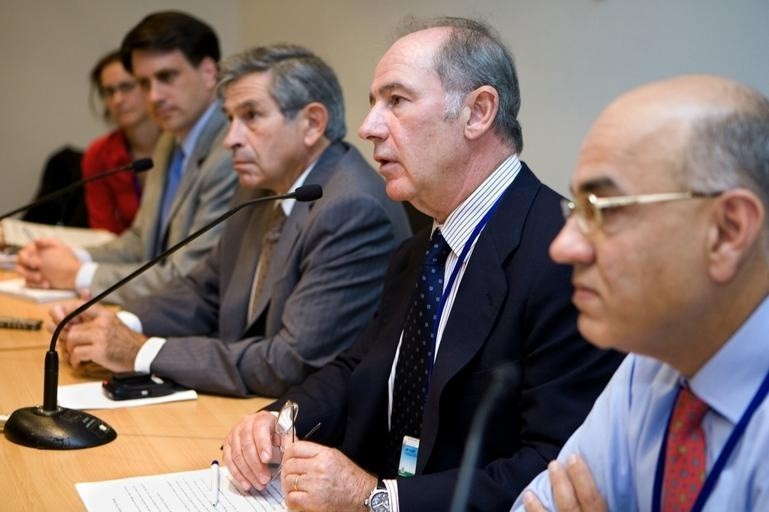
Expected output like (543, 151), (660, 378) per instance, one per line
(274, 398), (322, 458)
(559, 189), (723, 237)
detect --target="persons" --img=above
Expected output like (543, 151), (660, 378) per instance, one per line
(224, 17), (628, 512)
(46, 44), (413, 397)
(507, 75), (768, 512)
(79, 49), (164, 234)
(17, 11), (241, 306)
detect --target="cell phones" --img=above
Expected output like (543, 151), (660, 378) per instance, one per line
(102, 371), (177, 401)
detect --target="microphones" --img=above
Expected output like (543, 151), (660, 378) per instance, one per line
(0, 157), (154, 221)
(3, 183), (323, 450)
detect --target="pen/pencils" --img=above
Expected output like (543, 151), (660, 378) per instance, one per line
(210, 460), (219, 508)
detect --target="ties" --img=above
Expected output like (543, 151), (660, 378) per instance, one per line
(250, 203), (288, 321)
(389, 227), (452, 464)
(661, 387), (710, 511)
(156, 144), (184, 264)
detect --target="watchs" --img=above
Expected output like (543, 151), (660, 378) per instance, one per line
(365, 478), (389, 512)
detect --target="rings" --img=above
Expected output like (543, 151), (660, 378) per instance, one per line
(294, 474), (300, 491)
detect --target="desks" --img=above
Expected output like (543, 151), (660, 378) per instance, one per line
(0, 344), (300, 512)
(0, 265), (127, 351)
